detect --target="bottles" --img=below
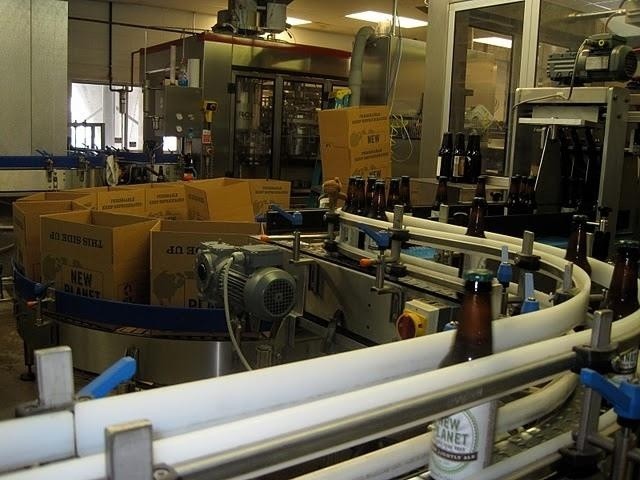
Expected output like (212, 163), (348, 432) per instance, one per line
(559, 184), (640, 414)
(157, 166), (164, 183)
(426, 269), (498, 479)
(436, 132), (483, 184)
(343, 175), (542, 239)
(184, 153), (193, 182)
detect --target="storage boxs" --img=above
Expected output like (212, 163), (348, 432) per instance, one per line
(319, 106), (393, 196)
(12, 176), (292, 308)
